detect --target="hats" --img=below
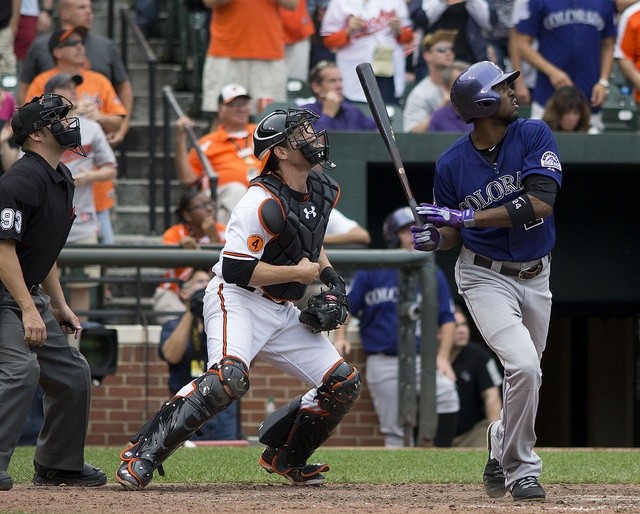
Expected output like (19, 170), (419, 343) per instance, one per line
(48, 26), (88, 49)
(11, 93), (87, 156)
(45, 73), (83, 92)
(177, 266), (209, 289)
(219, 83), (252, 106)
(422, 29), (458, 50)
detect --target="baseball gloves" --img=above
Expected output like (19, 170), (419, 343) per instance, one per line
(299, 276), (350, 334)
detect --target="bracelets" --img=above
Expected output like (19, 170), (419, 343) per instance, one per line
(597, 74), (612, 93)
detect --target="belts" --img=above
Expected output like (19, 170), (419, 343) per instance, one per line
(474, 254), (552, 282)
(28, 284), (45, 297)
(236, 284), (286, 305)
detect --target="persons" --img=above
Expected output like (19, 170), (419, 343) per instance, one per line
(513, 0), (616, 135)
(174, 84), (273, 220)
(312, 205), (373, 247)
(293, 60), (378, 130)
(20, 0), (135, 153)
(201, 0), (298, 114)
(31, 73), (120, 345)
(115, 106), (361, 486)
(538, 85), (590, 135)
(330, 203), (460, 449)
(617, 0), (640, 106)
(320, 1), (414, 102)
(9, 0), (41, 73)
(431, 63), (482, 135)
(411, 59), (565, 502)
(158, 265), (242, 443)
(402, 28), (472, 133)
(408, 0), (513, 81)
(22, 25), (129, 306)
(0, 91), (23, 175)
(278, 1), (317, 83)
(152, 183), (234, 324)
(0, 92), (109, 489)
(416, 303), (504, 449)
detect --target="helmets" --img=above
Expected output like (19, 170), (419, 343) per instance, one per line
(253, 107), (337, 172)
(450, 61), (520, 124)
(382, 207), (414, 249)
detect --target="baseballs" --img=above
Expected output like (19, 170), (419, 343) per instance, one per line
(185, 440), (191, 447)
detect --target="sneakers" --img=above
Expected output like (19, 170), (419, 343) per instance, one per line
(483, 422), (507, 498)
(115, 461), (142, 491)
(509, 475), (545, 501)
(259, 447), (327, 485)
(32, 459), (107, 485)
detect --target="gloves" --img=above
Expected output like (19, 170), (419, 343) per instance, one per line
(416, 203), (475, 229)
(411, 224), (442, 252)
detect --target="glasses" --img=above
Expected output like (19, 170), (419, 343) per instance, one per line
(60, 39), (86, 48)
(311, 59), (336, 78)
(189, 200), (211, 213)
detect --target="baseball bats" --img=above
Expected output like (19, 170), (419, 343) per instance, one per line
(183, 440), (250, 446)
(356, 63), (436, 250)
